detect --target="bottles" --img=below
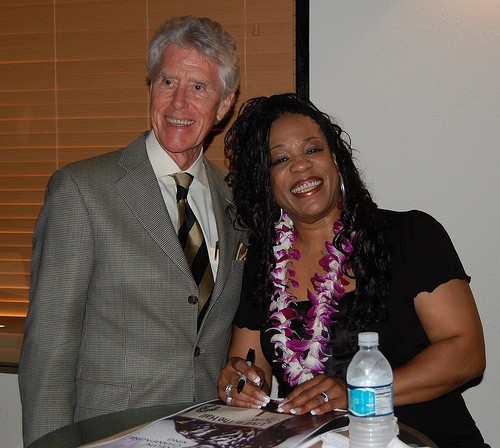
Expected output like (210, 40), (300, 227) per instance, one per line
(347, 332), (394, 448)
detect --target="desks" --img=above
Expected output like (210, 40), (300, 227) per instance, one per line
(28, 402), (437, 448)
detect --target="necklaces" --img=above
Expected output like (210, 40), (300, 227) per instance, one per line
(266, 203), (357, 387)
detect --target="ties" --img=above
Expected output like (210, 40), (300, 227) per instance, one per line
(170, 172), (215, 334)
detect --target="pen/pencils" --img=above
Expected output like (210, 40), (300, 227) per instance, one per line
(214, 241), (220, 260)
(236, 348), (255, 394)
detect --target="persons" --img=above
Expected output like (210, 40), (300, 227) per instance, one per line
(18, 16), (252, 448)
(218, 93), (491, 448)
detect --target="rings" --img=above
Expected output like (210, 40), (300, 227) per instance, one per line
(226, 397), (233, 406)
(321, 392), (329, 403)
(225, 384), (234, 396)
